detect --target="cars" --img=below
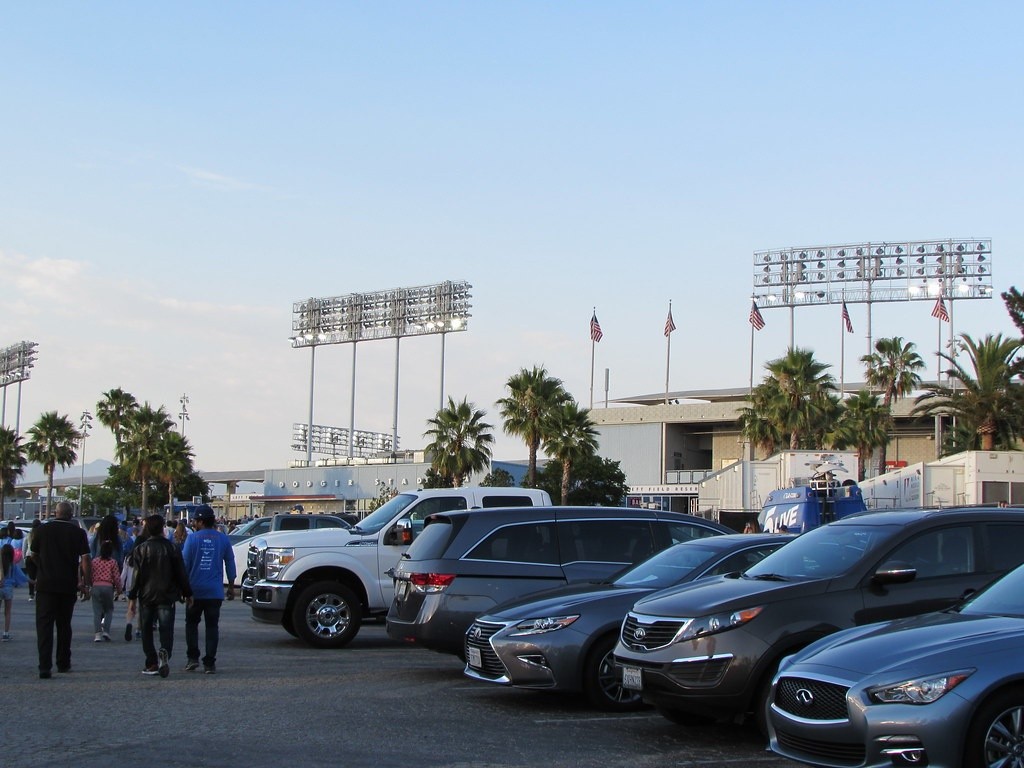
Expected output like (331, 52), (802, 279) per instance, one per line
(221, 517), (425, 587)
(764, 560), (1024, 768)
(0, 515), (104, 537)
(611, 503), (1024, 745)
(463, 531), (865, 715)
(384, 506), (772, 666)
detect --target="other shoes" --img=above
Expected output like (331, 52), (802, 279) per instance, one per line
(124, 623), (133, 642)
(58, 663), (72, 674)
(95, 631), (102, 641)
(135, 632), (140, 639)
(3, 635), (15, 642)
(102, 632), (110, 641)
(205, 665), (216, 673)
(185, 658), (201, 671)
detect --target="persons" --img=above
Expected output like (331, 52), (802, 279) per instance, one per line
(0, 501), (261, 679)
(744, 518), (761, 534)
(778, 525), (788, 534)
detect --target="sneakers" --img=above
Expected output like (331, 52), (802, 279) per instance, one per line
(141, 662), (159, 674)
(158, 647), (170, 678)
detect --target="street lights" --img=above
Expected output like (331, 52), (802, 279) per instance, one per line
(78, 407), (92, 517)
(291, 280), (473, 464)
(178, 393), (189, 439)
(754, 235), (994, 382)
(0, 340), (38, 470)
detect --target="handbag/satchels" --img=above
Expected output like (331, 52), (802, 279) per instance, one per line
(11, 549), (22, 563)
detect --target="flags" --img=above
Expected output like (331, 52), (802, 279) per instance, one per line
(664, 307), (676, 337)
(931, 293), (949, 322)
(842, 300), (854, 333)
(589, 315), (603, 343)
(748, 300), (766, 330)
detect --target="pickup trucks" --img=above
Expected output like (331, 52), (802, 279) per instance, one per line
(221, 514), (353, 547)
(241, 485), (554, 650)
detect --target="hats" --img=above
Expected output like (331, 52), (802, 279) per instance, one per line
(192, 504), (215, 519)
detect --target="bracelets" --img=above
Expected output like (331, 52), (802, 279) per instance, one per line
(85, 585), (91, 588)
